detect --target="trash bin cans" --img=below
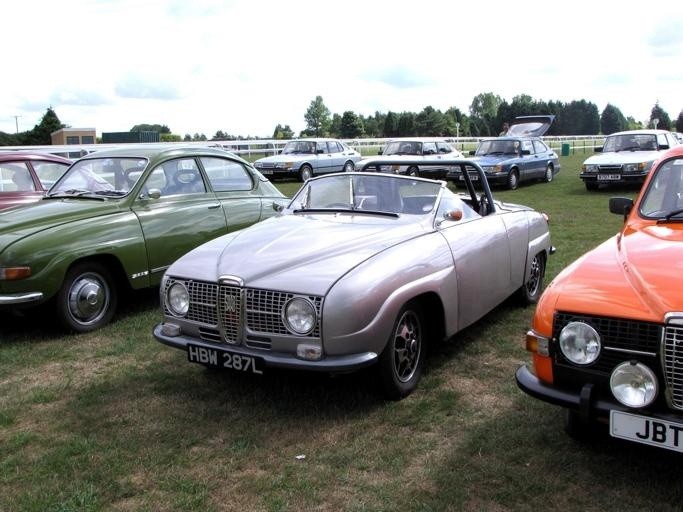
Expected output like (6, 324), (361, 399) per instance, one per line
(561, 144), (569, 156)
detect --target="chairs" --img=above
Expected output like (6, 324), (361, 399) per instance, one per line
(123, 166), (148, 195)
(167, 169), (204, 193)
(11, 171), (32, 192)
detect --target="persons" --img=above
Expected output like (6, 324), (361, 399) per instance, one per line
(499, 121), (519, 148)
